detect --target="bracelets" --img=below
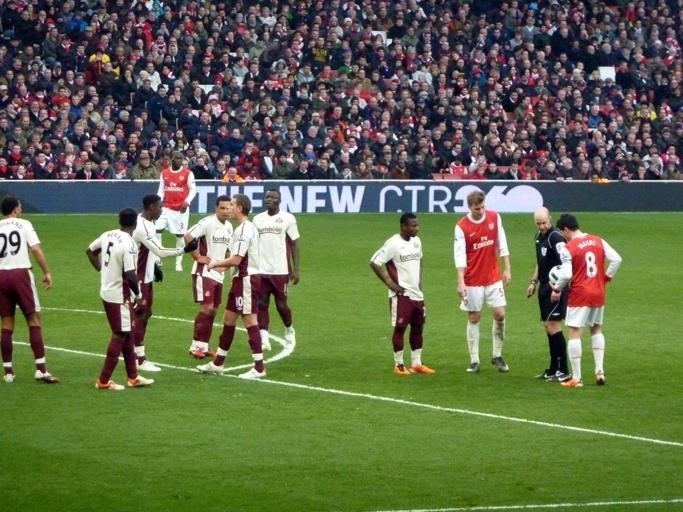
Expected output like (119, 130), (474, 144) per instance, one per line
(553, 288), (561, 294)
(531, 279), (538, 284)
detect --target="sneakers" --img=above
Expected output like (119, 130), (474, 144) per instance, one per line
(533, 368), (584, 387)
(189, 328), (295, 379)
(136, 359), (161, 371)
(95, 378), (124, 390)
(4, 374), (16, 382)
(154, 260), (163, 267)
(126, 376), (154, 387)
(394, 363), (410, 375)
(466, 363), (480, 372)
(176, 264), (183, 272)
(492, 357), (509, 372)
(594, 369), (605, 385)
(34, 370), (59, 383)
(409, 365), (435, 375)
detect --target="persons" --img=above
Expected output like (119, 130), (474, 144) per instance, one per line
(453, 189), (513, 373)
(556, 215), (623, 388)
(196, 194), (268, 381)
(156, 151), (198, 272)
(132, 193), (199, 373)
(85, 208), (155, 392)
(1, 0), (683, 184)
(526, 206), (573, 383)
(0, 196), (61, 384)
(369, 212), (437, 376)
(183, 195), (233, 359)
(252, 189), (301, 351)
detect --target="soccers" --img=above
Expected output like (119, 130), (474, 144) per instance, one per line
(549, 265), (562, 284)
